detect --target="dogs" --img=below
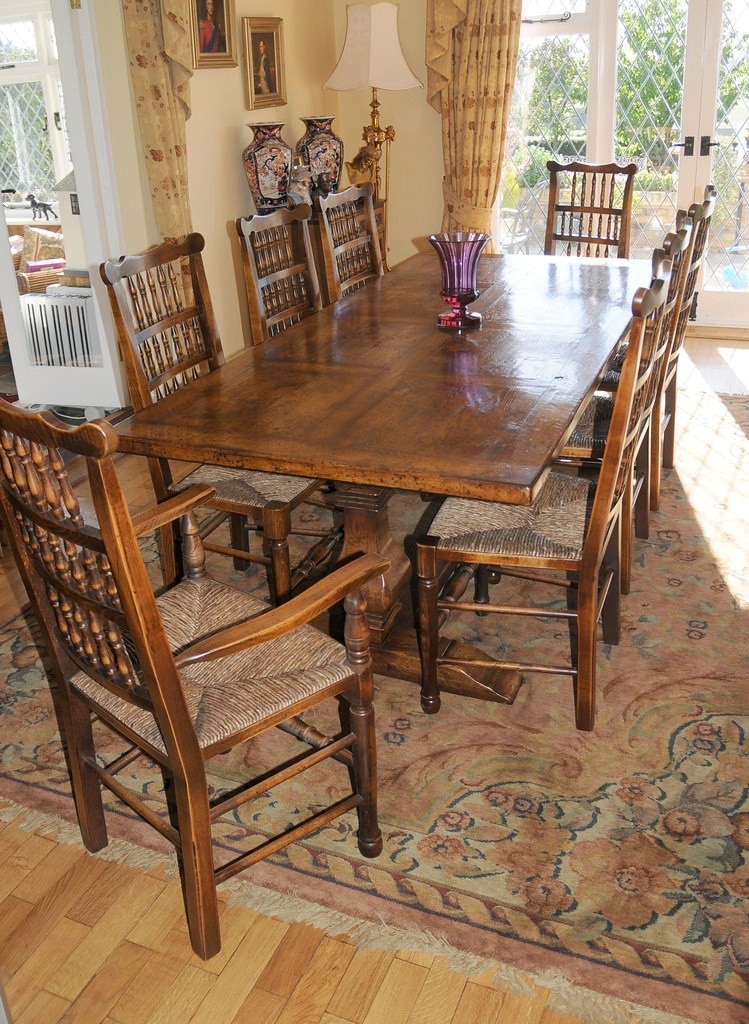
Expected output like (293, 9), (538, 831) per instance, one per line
(24, 194), (59, 221)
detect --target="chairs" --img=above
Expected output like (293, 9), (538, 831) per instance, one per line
(311, 181), (384, 310)
(499, 179), (550, 254)
(488, 216), (695, 594)
(99, 231), (346, 610)
(414, 253), (676, 733)
(596, 200), (711, 512)
(545, 159), (639, 258)
(0, 395), (392, 960)
(236, 204), (322, 345)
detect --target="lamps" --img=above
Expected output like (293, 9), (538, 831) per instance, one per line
(322, 2), (425, 270)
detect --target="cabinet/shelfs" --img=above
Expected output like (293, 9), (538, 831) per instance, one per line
(249, 198), (389, 307)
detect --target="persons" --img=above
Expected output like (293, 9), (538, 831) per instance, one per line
(288, 167), (314, 220)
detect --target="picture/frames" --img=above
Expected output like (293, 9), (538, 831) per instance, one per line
(188, 0), (239, 69)
(241, 16), (288, 110)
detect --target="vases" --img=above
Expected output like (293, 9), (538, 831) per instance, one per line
(241, 121), (293, 216)
(426, 231), (492, 328)
(295, 115), (344, 201)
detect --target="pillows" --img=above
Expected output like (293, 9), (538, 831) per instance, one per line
(19, 224), (65, 271)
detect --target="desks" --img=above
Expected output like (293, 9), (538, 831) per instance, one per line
(111, 253), (659, 707)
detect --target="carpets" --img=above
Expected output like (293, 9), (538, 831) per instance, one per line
(0, 387), (749, 1024)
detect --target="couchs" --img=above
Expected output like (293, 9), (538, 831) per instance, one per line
(0, 222), (67, 353)
